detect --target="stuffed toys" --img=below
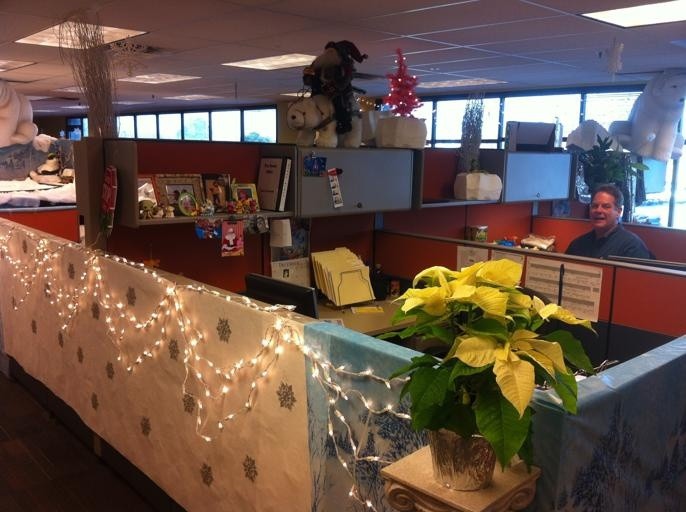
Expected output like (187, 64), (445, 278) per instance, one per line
(605, 68), (686, 162)
(302, 40), (369, 135)
(285, 95), (363, 150)
(137, 198), (156, 220)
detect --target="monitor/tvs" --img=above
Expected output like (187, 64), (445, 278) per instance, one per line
(246, 272), (319, 319)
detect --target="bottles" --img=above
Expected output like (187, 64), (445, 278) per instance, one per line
(311, 159), (319, 176)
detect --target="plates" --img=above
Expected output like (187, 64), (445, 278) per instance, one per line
(178, 192), (199, 216)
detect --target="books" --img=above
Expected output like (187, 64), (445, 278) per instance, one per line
(256, 154), (293, 211)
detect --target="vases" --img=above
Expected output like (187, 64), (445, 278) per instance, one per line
(425, 427), (498, 491)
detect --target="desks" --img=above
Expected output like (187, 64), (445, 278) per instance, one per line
(317, 295), (424, 335)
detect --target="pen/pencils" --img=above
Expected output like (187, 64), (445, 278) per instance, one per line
(227, 173), (236, 189)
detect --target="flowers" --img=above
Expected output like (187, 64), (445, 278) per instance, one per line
(375, 257), (599, 473)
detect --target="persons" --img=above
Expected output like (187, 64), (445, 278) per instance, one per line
(565, 184), (650, 266)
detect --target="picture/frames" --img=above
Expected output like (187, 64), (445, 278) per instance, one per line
(137, 173), (261, 212)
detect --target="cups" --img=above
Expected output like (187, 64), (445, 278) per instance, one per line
(498, 240), (513, 247)
